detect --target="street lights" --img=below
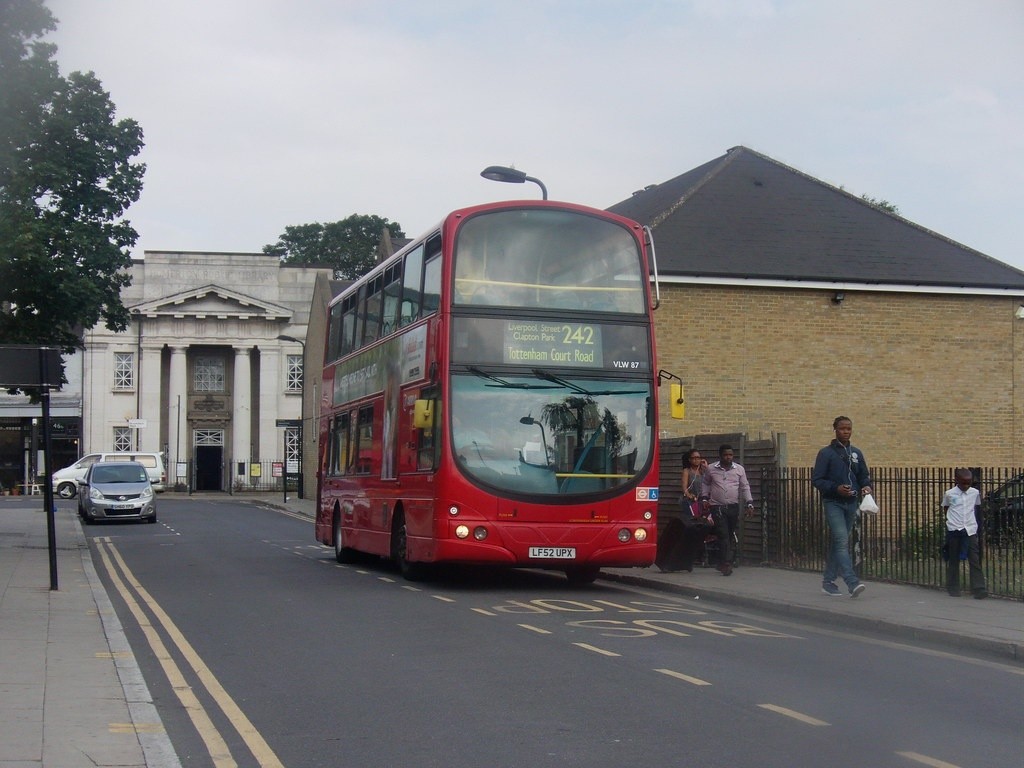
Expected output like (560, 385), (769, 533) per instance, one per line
(276, 335), (305, 498)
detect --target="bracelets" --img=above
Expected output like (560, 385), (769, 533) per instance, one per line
(703, 500), (707, 502)
(748, 504), (753, 508)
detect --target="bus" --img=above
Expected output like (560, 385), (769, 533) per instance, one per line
(313, 201), (686, 588)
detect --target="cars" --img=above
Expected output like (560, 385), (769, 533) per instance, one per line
(982, 473), (1024, 549)
(76, 460), (157, 525)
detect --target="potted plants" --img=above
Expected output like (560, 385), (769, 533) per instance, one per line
(3, 488), (10, 496)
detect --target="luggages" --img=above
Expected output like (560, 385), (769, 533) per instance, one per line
(654, 509), (711, 574)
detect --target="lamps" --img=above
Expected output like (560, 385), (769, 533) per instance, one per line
(831, 291), (845, 303)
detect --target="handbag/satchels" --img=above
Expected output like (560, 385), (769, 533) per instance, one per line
(859, 494), (879, 513)
(678, 494), (688, 505)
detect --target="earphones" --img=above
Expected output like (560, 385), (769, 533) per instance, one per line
(834, 430), (836, 433)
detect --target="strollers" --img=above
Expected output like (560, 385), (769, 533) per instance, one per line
(683, 495), (742, 568)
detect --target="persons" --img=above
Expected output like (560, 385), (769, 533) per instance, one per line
(678, 449), (708, 526)
(812, 416), (873, 597)
(940, 468), (989, 600)
(701, 443), (754, 574)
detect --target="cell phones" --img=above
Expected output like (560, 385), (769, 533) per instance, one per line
(843, 485), (849, 489)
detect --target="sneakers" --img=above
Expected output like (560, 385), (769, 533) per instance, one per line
(823, 582), (843, 596)
(850, 583), (865, 598)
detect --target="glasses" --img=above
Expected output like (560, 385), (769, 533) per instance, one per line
(689, 456), (700, 459)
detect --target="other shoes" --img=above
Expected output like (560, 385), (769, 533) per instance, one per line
(946, 585), (961, 597)
(718, 562), (733, 576)
(973, 588), (988, 599)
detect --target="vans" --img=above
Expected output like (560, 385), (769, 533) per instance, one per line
(51, 452), (169, 498)
